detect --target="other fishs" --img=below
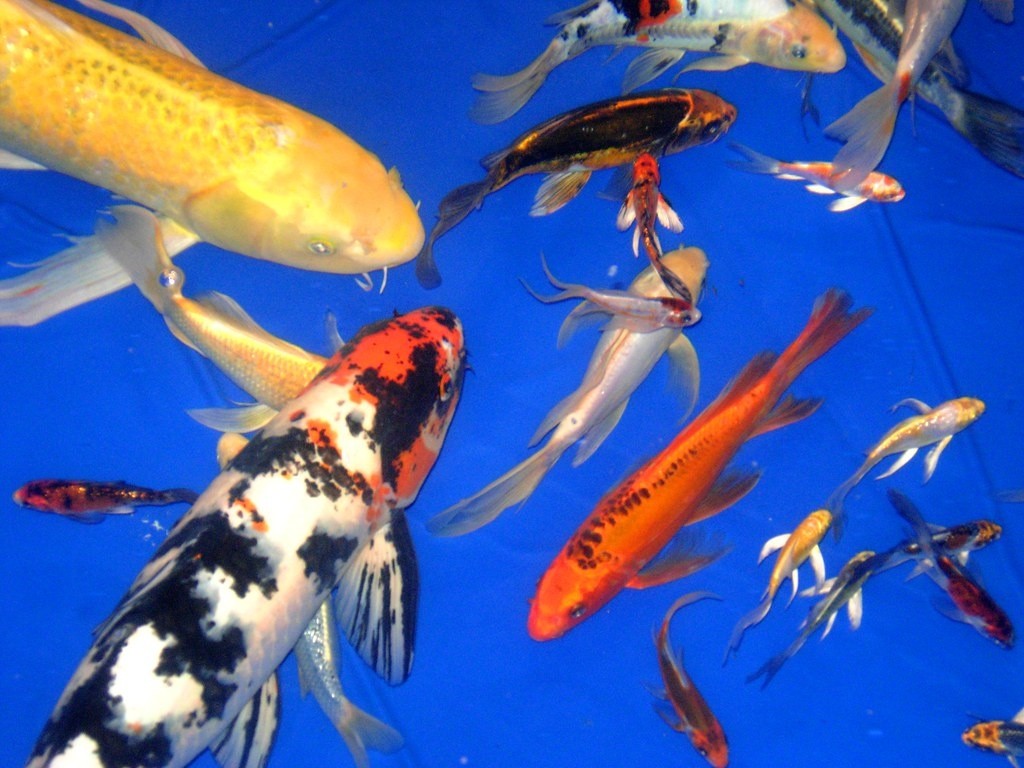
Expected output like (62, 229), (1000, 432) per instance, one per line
(1, 0), (1024, 762)
